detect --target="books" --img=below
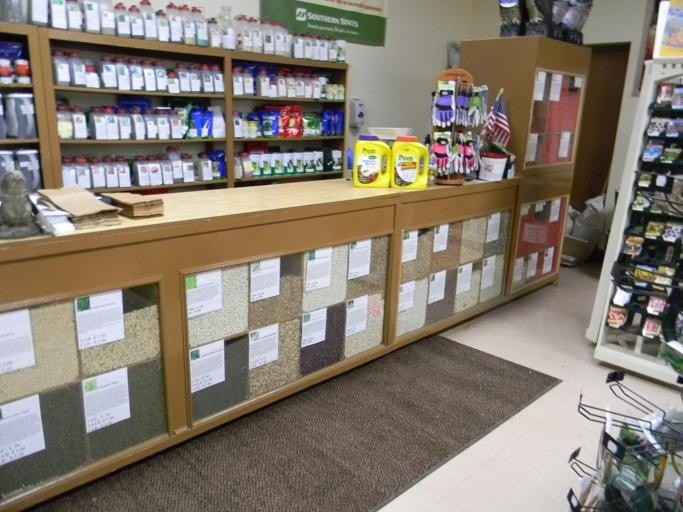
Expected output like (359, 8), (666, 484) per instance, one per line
(36, 185), (122, 238)
(102, 190), (164, 218)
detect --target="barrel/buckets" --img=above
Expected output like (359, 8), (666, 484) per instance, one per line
(480, 152), (508, 180)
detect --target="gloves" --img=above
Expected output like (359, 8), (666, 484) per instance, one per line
(429, 90), (487, 177)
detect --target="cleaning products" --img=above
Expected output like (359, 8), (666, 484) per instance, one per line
(353, 134), (390, 187)
(390, 135), (429, 190)
(0, 0), (346, 192)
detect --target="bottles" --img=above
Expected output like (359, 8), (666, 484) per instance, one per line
(48, 47), (226, 95)
(28, 1), (347, 66)
(232, 110), (244, 139)
(233, 150), (253, 179)
(60, 146), (214, 190)
(232, 66), (345, 101)
(55, 103), (183, 140)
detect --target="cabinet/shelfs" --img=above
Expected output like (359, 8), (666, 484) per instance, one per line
(0, 169), (572, 512)
(459, 36), (593, 179)
(585, 58), (683, 389)
(0, 21), (350, 193)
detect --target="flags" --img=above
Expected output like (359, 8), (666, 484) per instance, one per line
(482, 96), (511, 147)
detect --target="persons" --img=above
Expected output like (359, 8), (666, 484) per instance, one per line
(0, 171), (39, 239)
